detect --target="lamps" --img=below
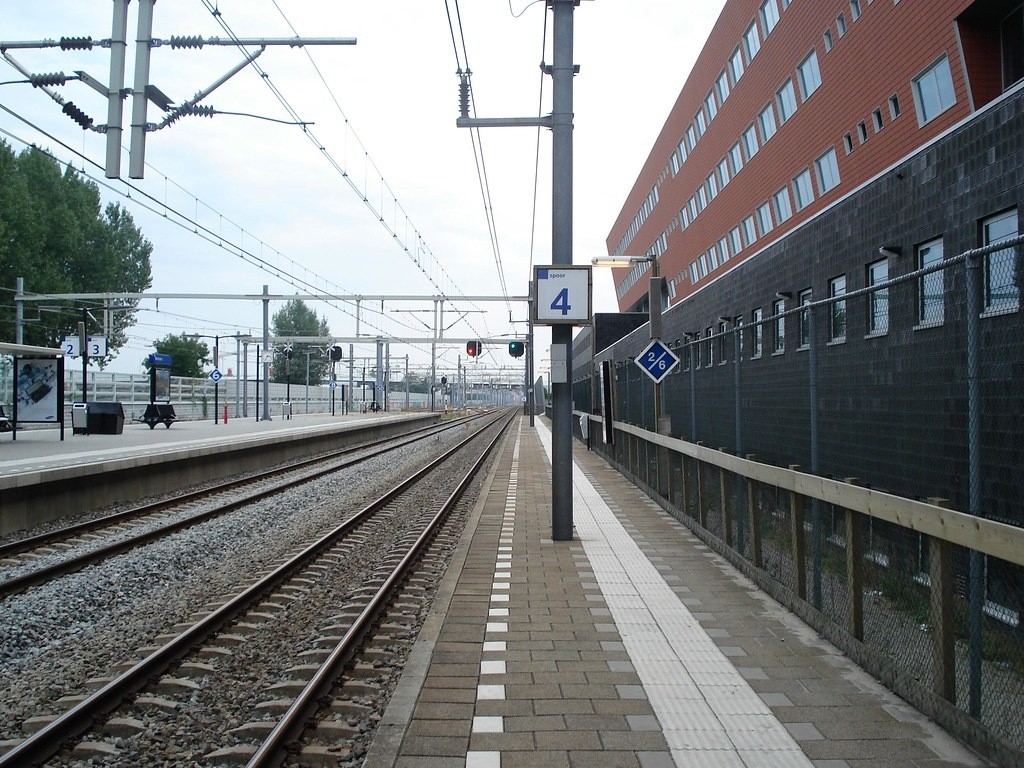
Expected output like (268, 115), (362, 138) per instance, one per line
(775, 291), (792, 300)
(878, 247), (901, 258)
(683, 332), (693, 338)
(720, 317), (731, 322)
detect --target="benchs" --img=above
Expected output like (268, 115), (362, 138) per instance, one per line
(134, 403), (188, 430)
(368, 402), (382, 413)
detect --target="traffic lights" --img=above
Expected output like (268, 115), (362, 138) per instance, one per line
(509, 342), (524, 357)
(467, 342), (481, 357)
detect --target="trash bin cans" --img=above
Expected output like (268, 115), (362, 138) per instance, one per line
(69, 402), (125, 436)
